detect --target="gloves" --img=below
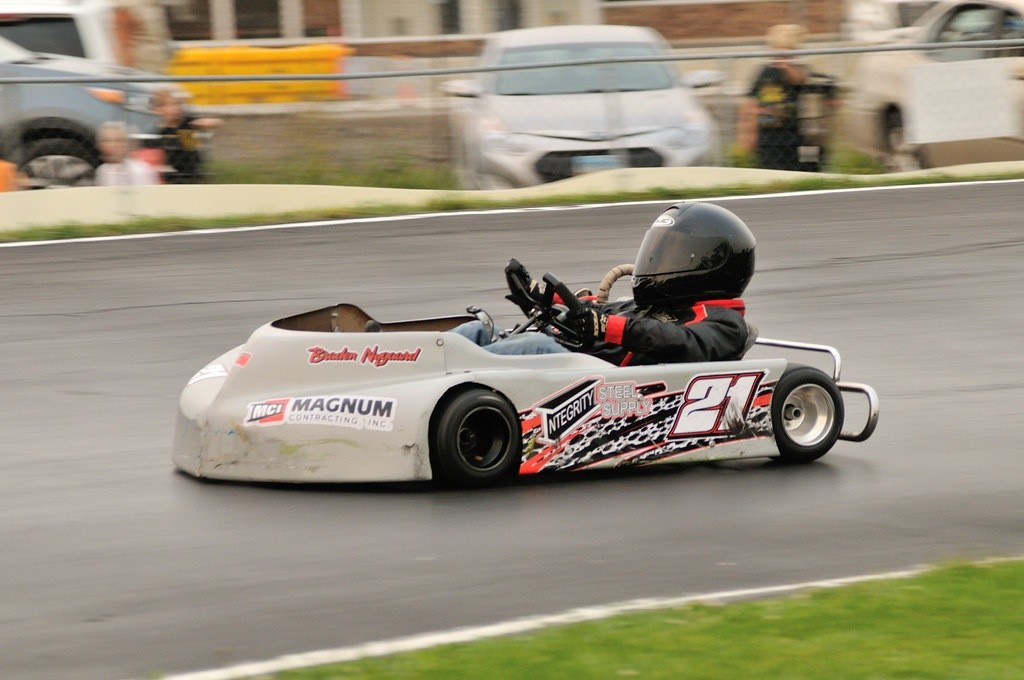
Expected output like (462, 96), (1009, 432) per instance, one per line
(566, 307), (631, 344)
(504, 258), (565, 306)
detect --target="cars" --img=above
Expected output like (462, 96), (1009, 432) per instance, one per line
(1, 34), (201, 192)
(0, 1), (169, 79)
(832, 2), (1024, 162)
(439, 24), (728, 190)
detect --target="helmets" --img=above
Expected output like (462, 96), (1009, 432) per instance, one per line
(632, 201), (756, 307)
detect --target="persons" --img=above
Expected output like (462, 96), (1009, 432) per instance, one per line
(732, 27), (811, 168)
(146, 90), (226, 182)
(92, 121), (162, 186)
(445, 198), (755, 369)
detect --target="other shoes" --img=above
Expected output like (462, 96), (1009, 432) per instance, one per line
(364, 319), (383, 333)
